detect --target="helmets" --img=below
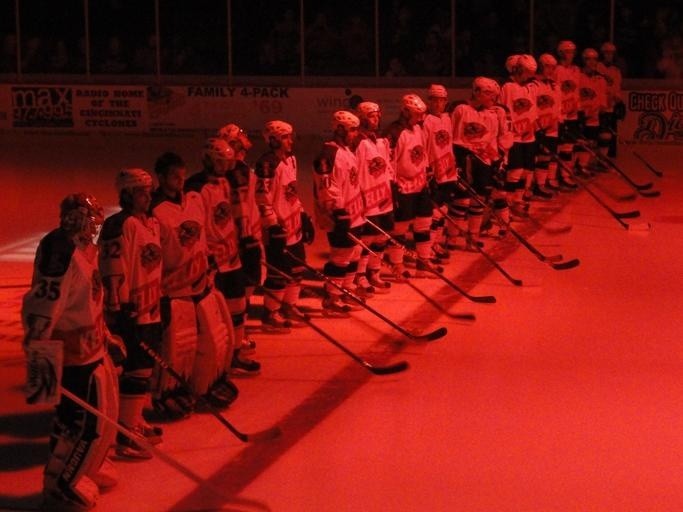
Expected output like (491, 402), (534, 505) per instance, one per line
(400, 93), (427, 115)
(217, 124), (251, 152)
(540, 54), (558, 67)
(115, 169), (153, 197)
(59, 192), (102, 227)
(330, 110), (360, 132)
(427, 84), (448, 98)
(358, 102), (380, 120)
(203, 136), (235, 161)
(472, 77), (499, 96)
(583, 48), (598, 58)
(506, 54), (520, 74)
(557, 40), (577, 50)
(262, 120), (293, 149)
(517, 54), (537, 73)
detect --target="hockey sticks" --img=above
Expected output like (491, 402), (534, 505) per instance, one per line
(57, 388), (270, 511)
(611, 132), (664, 177)
(549, 149), (652, 229)
(366, 220), (496, 304)
(591, 149), (662, 198)
(136, 343), (281, 439)
(240, 272), (411, 373)
(348, 231), (476, 321)
(461, 187), (580, 269)
(571, 167), (636, 203)
(288, 253), (448, 341)
(430, 202), (523, 286)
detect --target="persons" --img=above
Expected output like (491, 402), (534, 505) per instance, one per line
(353, 101), (395, 294)
(312, 108), (367, 318)
(20, 192), (121, 512)
(421, 42), (624, 265)
(98, 166), (162, 460)
(149, 149), (239, 419)
(182, 136), (262, 373)
(258, 118), (314, 330)
(384, 94), (445, 275)
(219, 122), (267, 331)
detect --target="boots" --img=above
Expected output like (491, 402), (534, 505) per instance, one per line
(227, 331), (259, 356)
(364, 268), (392, 294)
(320, 290), (351, 320)
(107, 419), (154, 460)
(280, 296), (311, 326)
(426, 250), (442, 266)
(259, 299), (295, 335)
(498, 224), (510, 241)
(139, 410), (163, 444)
(217, 354), (263, 380)
(354, 269), (375, 295)
(413, 259), (444, 278)
(463, 237), (485, 253)
(341, 283), (368, 308)
(431, 240), (451, 261)
(388, 263), (411, 281)
